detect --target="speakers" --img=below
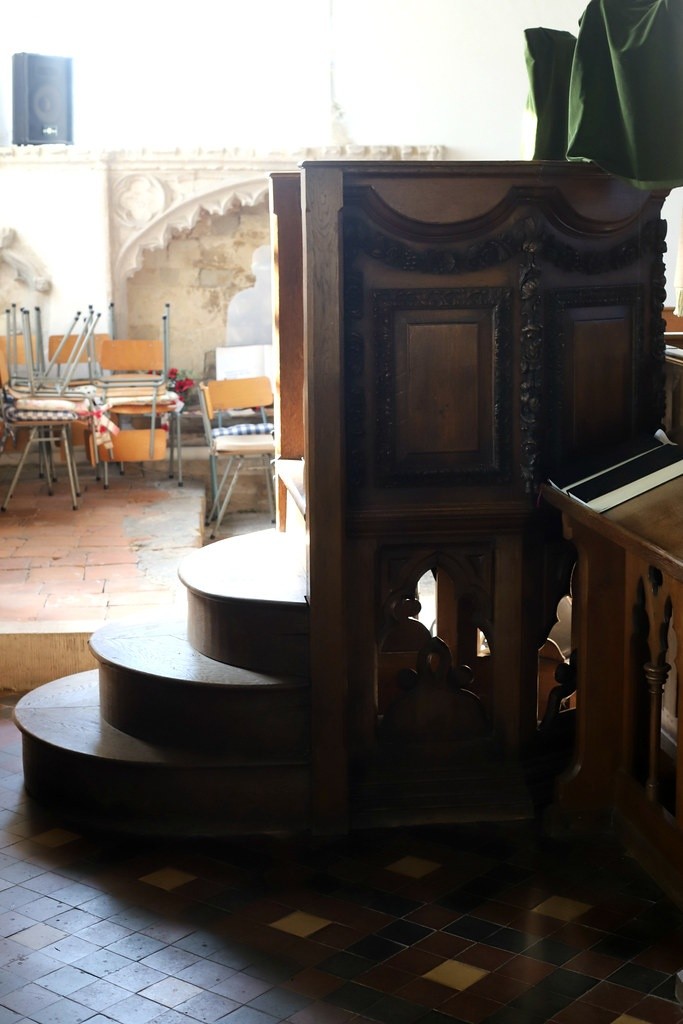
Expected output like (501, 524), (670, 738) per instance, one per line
(11, 53), (73, 145)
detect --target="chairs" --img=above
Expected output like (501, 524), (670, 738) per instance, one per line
(0, 302), (276, 539)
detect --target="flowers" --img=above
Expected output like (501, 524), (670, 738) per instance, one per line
(149, 367), (195, 409)
